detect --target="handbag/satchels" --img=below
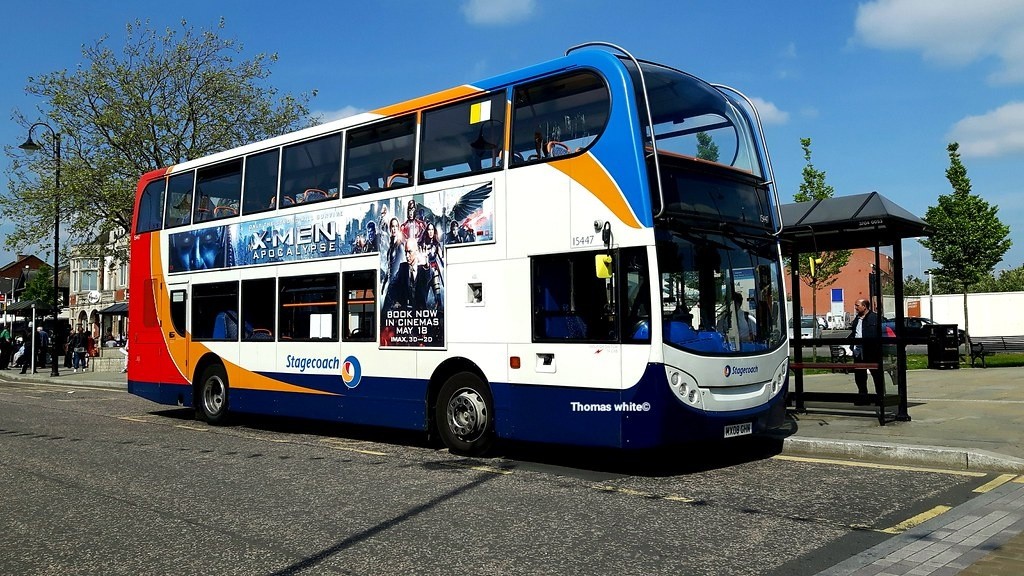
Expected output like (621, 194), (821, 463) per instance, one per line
(0, 336), (12, 349)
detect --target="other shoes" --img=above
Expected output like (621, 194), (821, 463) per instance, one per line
(74, 368), (77, 373)
(34, 371), (38, 373)
(82, 369), (85, 373)
(3, 367), (11, 370)
(19, 372), (26, 374)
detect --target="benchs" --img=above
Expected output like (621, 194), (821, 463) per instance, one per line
(967, 336), (1024, 369)
(829, 345), (854, 374)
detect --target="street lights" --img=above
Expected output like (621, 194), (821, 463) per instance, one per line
(924, 269), (934, 326)
(17, 123), (63, 378)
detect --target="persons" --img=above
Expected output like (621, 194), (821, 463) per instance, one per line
(847, 298), (887, 406)
(0, 321), (128, 374)
(717, 291), (756, 343)
(352, 202), (475, 346)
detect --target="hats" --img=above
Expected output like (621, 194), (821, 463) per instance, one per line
(28, 322), (32, 327)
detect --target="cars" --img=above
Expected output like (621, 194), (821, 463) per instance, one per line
(807, 315), (828, 330)
(878, 316), (896, 336)
(888, 317), (971, 345)
(786, 315), (822, 346)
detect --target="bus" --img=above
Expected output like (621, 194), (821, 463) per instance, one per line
(127, 40), (800, 460)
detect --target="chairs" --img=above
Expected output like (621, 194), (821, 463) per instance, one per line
(499, 141), (585, 165)
(250, 329), (273, 339)
(196, 173), (410, 221)
(352, 328), (370, 339)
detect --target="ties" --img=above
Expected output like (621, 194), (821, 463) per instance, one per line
(411, 270), (415, 294)
